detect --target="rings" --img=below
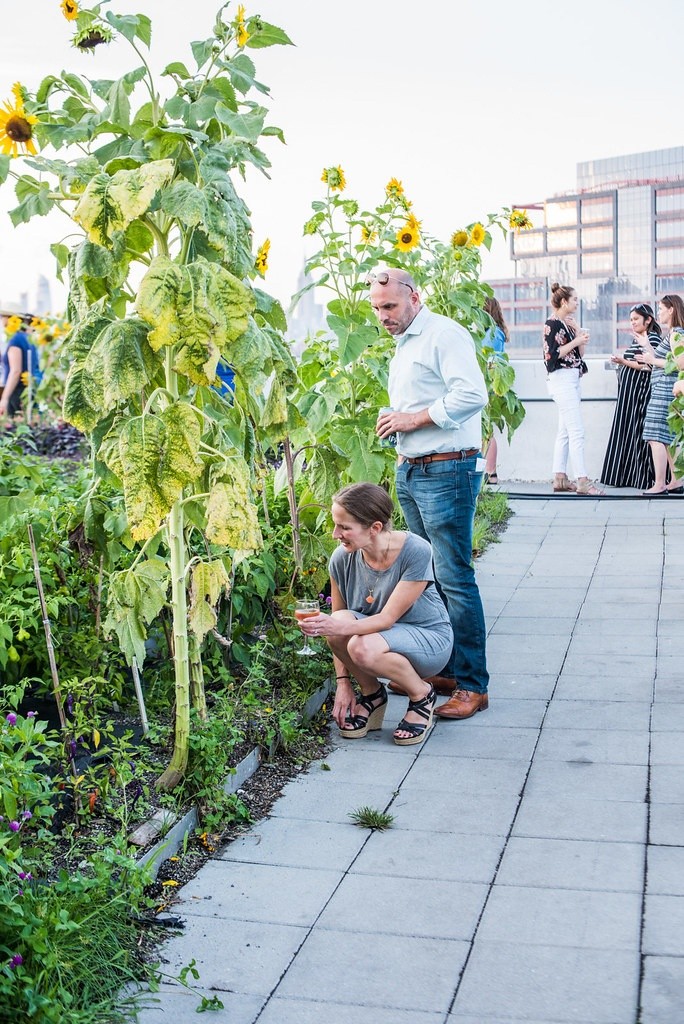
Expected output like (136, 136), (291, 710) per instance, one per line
(315, 629), (317, 634)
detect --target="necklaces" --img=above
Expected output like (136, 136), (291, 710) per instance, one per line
(364, 531), (392, 604)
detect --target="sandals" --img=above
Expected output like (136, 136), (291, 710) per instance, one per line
(577, 478), (606, 496)
(552, 476), (577, 492)
(485, 473), (497, 484)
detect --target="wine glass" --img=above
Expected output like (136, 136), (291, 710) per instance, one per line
(294, 599), (320, 655)
(640, 349), (652, 371)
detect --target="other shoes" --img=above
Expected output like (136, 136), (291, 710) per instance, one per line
(667, 486), (684, 494)
(643, 488), (668, 495)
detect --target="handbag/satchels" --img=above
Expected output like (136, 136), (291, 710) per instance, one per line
(581, 362), (587, 373)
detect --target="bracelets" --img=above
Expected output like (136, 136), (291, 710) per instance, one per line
(336, 675), (351, 680)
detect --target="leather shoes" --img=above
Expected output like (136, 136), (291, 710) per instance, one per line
(432, 688), (488, 719)
(386, 673), (457, 696)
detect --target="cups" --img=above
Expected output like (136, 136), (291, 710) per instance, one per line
(579, 328), (590, 345)
(611, 355), (620, 369)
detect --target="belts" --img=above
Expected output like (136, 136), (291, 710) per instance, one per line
(407, 450), (479, 464)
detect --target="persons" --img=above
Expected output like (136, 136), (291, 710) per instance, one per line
(480, 295), (509, 485)
(298, 482), (454, 746)
(370, 268), (489, 719)
(631, 294), (684, 496)
(600, 303), (672, 491)
(0, 300), (40, 421)
(542, 283), (606, 496)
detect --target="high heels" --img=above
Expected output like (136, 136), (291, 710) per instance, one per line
(393, 680), (437, 746)
(338, 682), (388, 739)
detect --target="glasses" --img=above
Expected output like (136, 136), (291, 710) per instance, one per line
(662, 294), (673, 306)
(631, 303), (649, 313)
(365, 272), (413, 293)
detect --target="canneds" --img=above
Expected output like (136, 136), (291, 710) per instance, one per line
(378, 406), (397, 447)
